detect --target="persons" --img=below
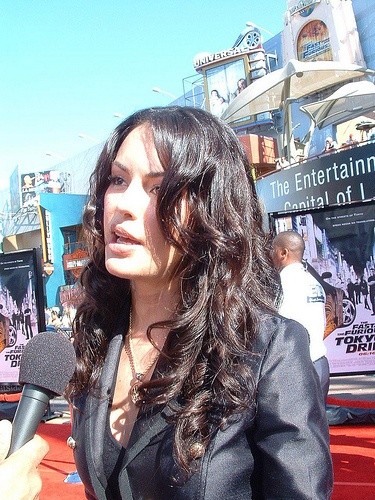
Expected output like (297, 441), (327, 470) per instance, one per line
(65, 106), (336, 500)
(12, 303), (34, 340)
(320, 134), (357, 156)
(276, 156), (290, 171)
(209, 79), (246, 117)
(45, 304), (78, 328)
(23, 173), (56, 201)
(270, 231), (330, 416)
(345, 269), (375, 316)
(1, 419), (51, 500)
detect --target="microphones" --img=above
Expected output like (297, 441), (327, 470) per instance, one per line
(6, 332), (77, 460)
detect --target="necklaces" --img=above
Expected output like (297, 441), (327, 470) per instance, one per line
(128, 306), (174, 405)
